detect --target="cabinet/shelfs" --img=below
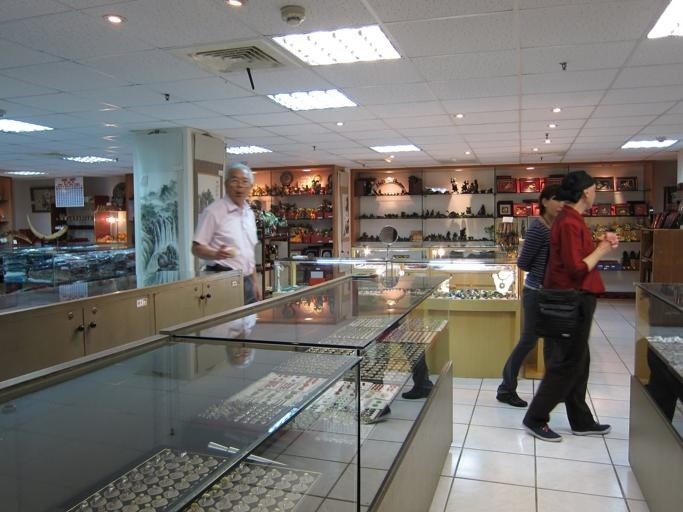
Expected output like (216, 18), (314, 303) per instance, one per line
(245, 163), (349, 324)
(0, 331), (366, 512)
(0, 176), (14, 243)
(350, 239), (525, 258)
(628, 283), (682, 511)
(0, 269), (252, 378)
(494, 159), (658, 272)
(93, 207), (127, 244)
(349, 162), (494, 259)
(268, 258), (522, 380)
(0, 245), (135, 281)
(160, 271), (453, 512)
(638, 229), (681, 282)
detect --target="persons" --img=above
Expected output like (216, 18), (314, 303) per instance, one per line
(194, 161), (261, 305)
(520, 170), (619, 442)
(356, 277), (434, 422)
(200, 312), (261, 367)
(494, 184), (569, 408)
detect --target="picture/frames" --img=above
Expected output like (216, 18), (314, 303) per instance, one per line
(29, 185), (63, 212)
(51, 192), (110, 248)
(126, 172), (135, 246)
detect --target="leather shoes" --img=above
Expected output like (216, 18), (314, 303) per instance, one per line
(400, 384), (434, 398)
(495, 390), (529, 406)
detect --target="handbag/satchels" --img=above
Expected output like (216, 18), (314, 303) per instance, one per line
(534, 243), (582, 344)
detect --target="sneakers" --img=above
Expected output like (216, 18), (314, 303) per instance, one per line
(570, 416), (612, 436)
(520, 418), (563, 442)
(354, 396), (392, 423)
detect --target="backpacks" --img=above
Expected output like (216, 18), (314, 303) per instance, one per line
(530, 215), (587, 343)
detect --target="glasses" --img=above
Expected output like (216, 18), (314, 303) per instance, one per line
(228, 179), (252, 188)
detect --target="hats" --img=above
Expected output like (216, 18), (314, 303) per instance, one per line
(552, 170), (595, 204)
